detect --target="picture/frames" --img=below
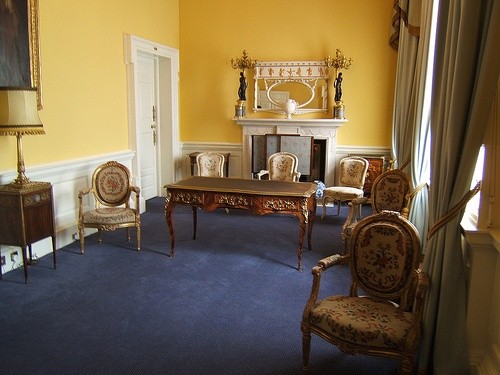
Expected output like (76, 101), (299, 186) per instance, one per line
(0, 0), (42, 111)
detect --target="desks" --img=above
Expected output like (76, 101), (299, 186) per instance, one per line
(189, 152), (230, 178)
(163, 175), (320, 272)
(347, 152), (390, 191)
(0, 185), (57, 283)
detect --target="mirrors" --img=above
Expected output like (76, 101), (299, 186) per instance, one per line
(253, 60), (329, 113)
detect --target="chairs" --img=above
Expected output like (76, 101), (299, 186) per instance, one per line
(300, 209), (431, 375)
(341, 169), (414, 256)
(196, 151), (224, 178)
(256, 151), (301, 183)
(76, 161), (140, 254)
(320, 157), (370, 220)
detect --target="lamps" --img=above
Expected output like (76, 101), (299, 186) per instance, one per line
(0, 86), (48, 189)
